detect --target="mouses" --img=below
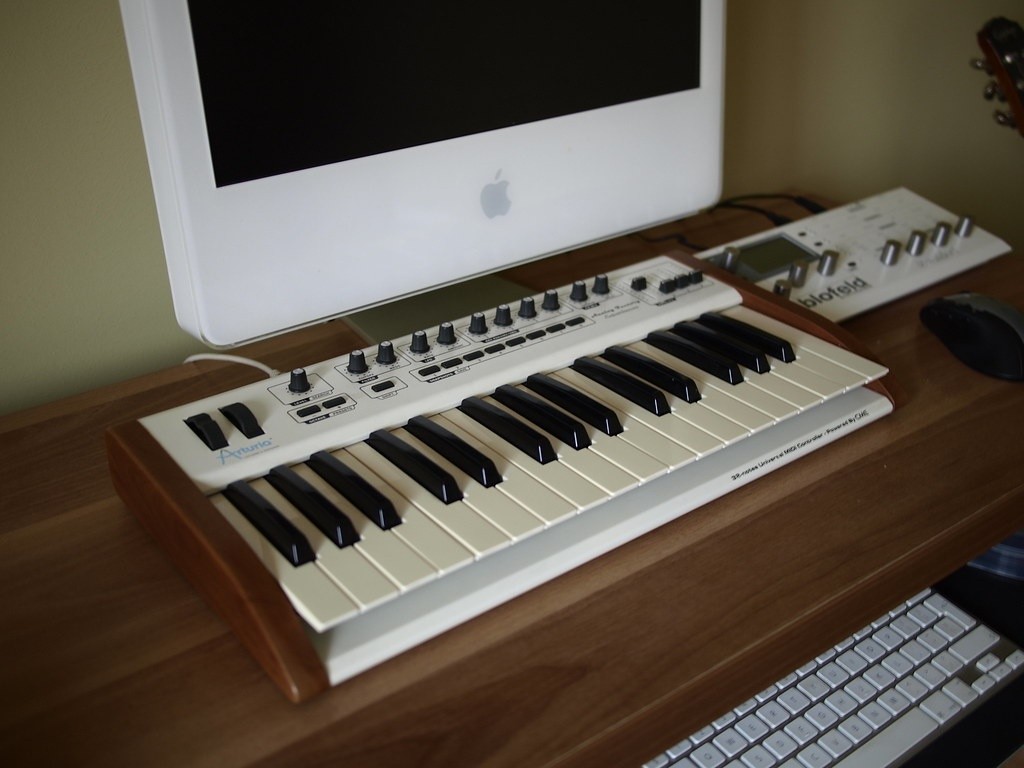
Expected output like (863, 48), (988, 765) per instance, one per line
(919, 291), (1024, 381)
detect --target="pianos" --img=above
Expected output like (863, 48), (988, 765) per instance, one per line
(98, 238), (902, 709)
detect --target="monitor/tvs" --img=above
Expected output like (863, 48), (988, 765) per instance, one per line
(118, 0), (726, 349)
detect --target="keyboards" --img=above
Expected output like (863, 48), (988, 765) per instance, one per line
(639, 586), (1024, 768)
